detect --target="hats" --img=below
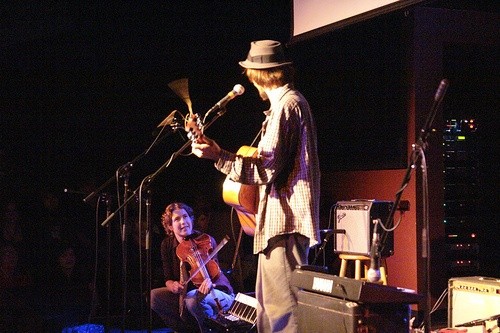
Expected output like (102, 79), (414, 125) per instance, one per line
(238, 40), (292, 69)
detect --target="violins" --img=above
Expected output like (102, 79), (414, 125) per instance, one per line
(176, 233), (228, 316)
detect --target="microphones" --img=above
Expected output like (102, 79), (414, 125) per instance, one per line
(213, 83), (245, 111)
(367, 222), (381, 282)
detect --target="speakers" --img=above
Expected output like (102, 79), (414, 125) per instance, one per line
(446, 276), (500, 333)
(295, 290), (410, 333)
(333, 201), (394, 256)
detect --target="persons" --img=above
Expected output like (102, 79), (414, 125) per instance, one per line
(190, 40), (321, 332)
(0, 180), (155, 333)
(146, 202), (235, 333)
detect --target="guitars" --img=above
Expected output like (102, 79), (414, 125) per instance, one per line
(184, 112), (260, 235)
